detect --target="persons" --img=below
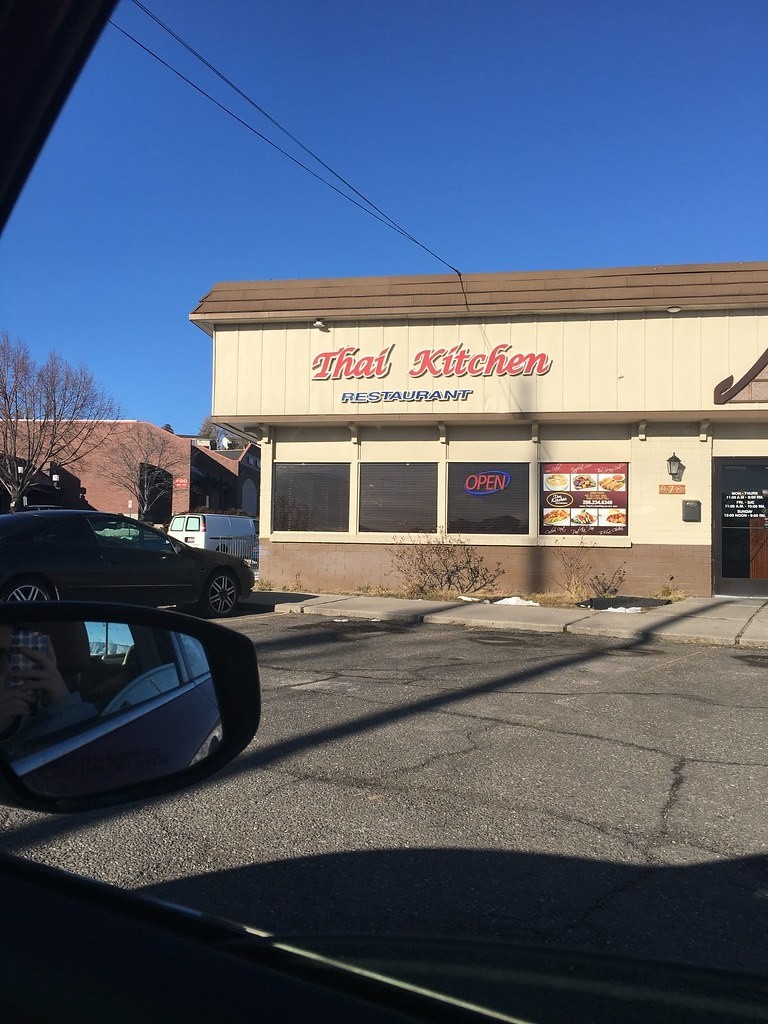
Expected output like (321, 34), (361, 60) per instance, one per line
(0, 624), (72, 762)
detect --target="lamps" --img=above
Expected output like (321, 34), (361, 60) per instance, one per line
(667, 452), (681, 476)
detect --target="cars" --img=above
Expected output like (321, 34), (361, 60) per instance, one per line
(1, 506), (254, 620)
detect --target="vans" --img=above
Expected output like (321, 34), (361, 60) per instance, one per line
(166, 512), (260, 559)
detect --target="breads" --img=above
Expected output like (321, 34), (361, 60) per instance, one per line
(599, 477), (624, 490)
